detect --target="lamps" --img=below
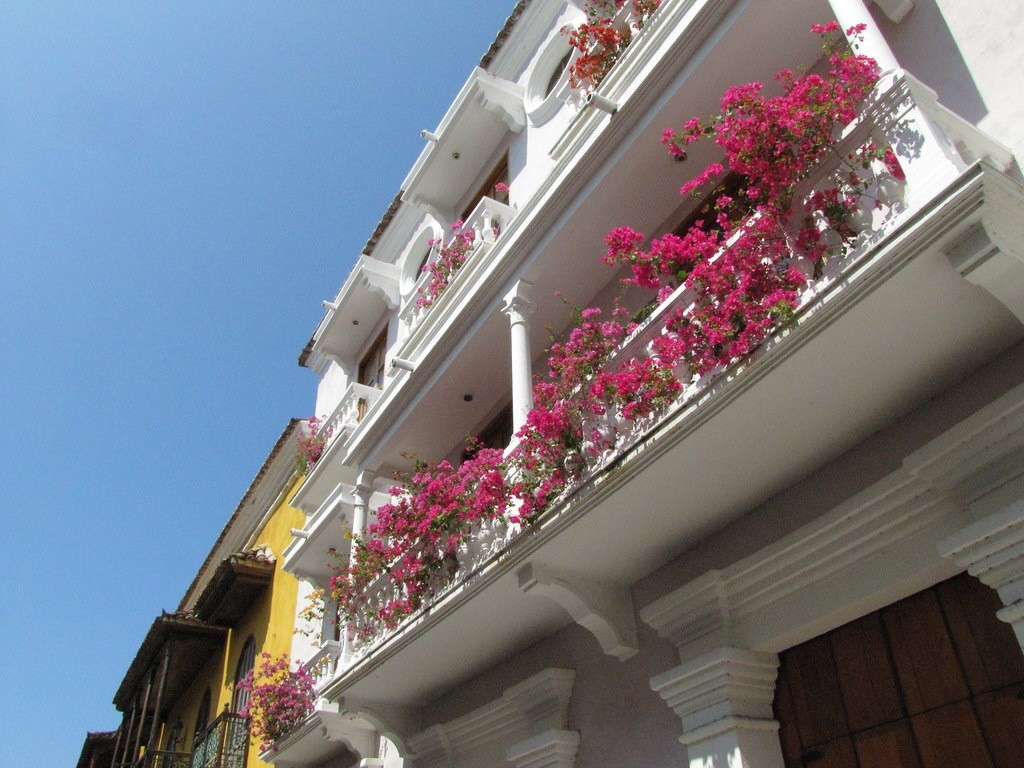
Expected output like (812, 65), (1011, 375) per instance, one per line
(172, 717), (186, 743)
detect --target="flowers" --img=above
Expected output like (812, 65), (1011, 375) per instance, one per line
(234, 0), (904, 755)
